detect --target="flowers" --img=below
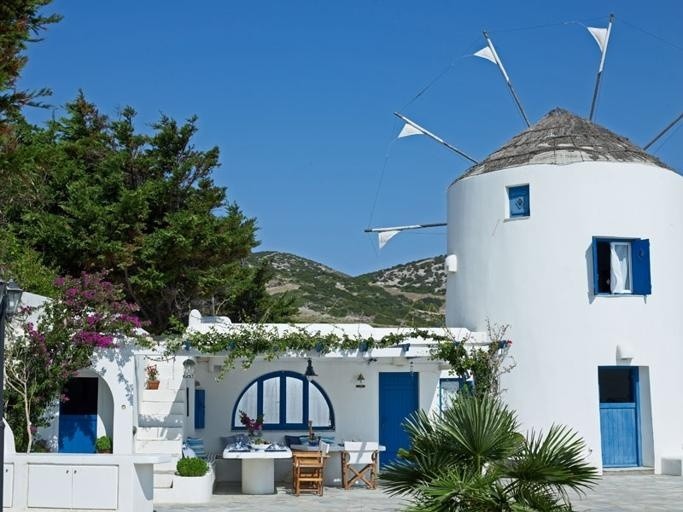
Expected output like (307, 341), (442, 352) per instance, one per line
(146, 364), (160, 381)
(237, 408), (265, 444)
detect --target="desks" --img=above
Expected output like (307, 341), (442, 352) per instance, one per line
(290, 444), (387, 452)
(223, 442), (293, 495)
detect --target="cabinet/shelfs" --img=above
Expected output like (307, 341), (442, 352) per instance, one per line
(2, 463), (14, 508)
(26, 464), (119, 511)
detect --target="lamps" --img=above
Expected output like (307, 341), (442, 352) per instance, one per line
(355, 373), (365, 389)
(304, 358), (319, 383)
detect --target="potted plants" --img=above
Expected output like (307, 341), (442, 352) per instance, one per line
(94, 436), (112, 453)
(172, 456), (215, 504)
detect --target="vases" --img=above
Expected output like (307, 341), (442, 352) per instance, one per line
(247, 430), (263, 445)
(147, 380), (160, 390)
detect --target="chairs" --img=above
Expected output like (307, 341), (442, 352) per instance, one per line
(292, 440), (331, 498)
(340, 440), (379, 490)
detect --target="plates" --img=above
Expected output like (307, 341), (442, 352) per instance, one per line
(247, 442), (272, 449)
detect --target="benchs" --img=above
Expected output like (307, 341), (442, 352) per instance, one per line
(216, 448), (371, 487)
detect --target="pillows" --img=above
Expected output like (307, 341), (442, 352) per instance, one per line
(182, 436), (208, 462)
(221, 433), (246, 447)
(285, 435), (321, 448)
